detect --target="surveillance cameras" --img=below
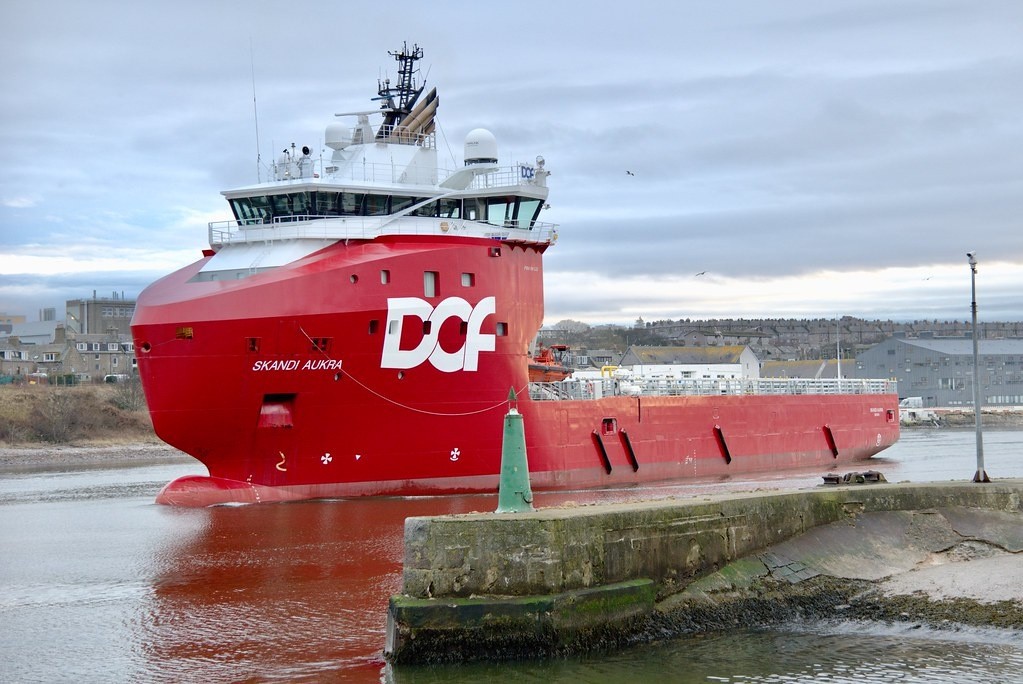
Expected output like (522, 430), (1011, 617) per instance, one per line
(966, 249), (976, 258)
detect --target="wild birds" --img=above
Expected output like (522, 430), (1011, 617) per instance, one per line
(626, 171), (634, 176)
(696, 271), (706, 276)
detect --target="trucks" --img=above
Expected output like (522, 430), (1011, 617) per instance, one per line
(899, 397), (922, 407)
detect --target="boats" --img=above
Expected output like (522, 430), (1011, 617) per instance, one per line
(128, 36), (900, 508)
(527, 345), (575, 383)
